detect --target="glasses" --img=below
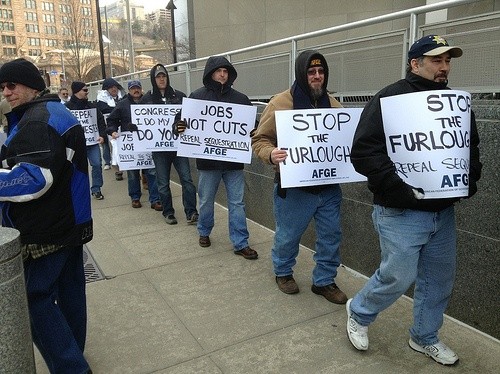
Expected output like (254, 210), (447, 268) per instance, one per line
(130, 85), (140, 90)
(0, 81), (21, 92)
(60, 92), (68, 95)
(81, 89), (89, 92)
(308, 68), (325, 76)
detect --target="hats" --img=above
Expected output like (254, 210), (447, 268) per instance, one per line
(408, 34), (463, 58)
(308, 54), (324, 68)
(155, 66), (167, 78)
(127, 80), (142, 90)
(71, 81), (86, 94)
(0, 58), (46, 91)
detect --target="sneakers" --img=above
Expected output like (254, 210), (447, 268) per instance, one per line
(199, 234), (211, 247)
(408, 336), (459, 366)
(345, 297), (369, 351)
(275, 274), (299, 294)
(186, 211), (200, 222)
(311, 283), (348, 305)
(131, 199), (141, 208)
(164, 213), (177, 224)
(150, 201), (163, 211)
(234, 246), (258, 259)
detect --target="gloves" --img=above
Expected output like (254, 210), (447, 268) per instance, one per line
(127, 123), (138, 132)
(387, 182), (414, 197)
(172, 121), (187, 134)
(468, 175), (478, 198)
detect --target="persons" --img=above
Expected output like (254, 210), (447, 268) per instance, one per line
(345, 35), (483, 367)
(0, 97), (11, 133)
(92, 78), (126, 170)
(125, 63), (199, 225)
(251, 50), (349, 304)
(64, 81), (105, 200)
(0, 56), (94, 374)
(106, 80), (164, 211)
(171, 55), (260, 259)
(58, 88), (71, 104)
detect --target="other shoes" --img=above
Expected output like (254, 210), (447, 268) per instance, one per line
(91, 191), (104, 200)
(104, 165), (149, 190)
